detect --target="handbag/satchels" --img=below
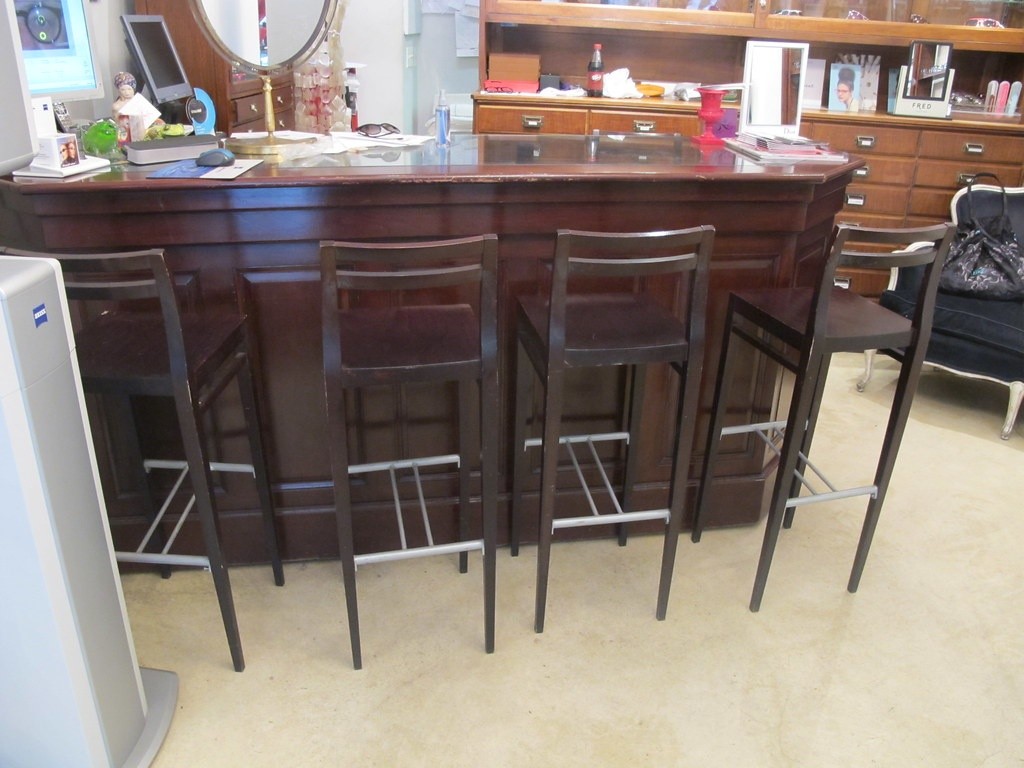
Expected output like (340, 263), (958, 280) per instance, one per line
(939, 172), (1024, 300)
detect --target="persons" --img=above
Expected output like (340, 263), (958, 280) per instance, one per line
(59, 142), (78, 168)
(837, 68), (858, 112)
(112, 71), (137, 142)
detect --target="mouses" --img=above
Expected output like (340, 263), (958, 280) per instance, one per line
(196, 149), (238, 168)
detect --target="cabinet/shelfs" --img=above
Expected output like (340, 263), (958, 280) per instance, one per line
(228, 70), (348, 134)
(478, 0), (1024, 304)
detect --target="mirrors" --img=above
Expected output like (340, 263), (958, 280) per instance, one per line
(197, 0), (326, 67)
(891, 40), (955, 117)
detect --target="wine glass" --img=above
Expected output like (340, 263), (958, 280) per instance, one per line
(692, 88), (729, 143)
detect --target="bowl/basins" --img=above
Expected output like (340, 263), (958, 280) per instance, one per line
(81, 125), (119, 154)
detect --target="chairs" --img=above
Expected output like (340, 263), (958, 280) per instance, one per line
(857, 183), (1024, 440)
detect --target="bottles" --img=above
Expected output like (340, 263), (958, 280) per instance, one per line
(435, 88), (451, 148)
(588, 43), (604, 97)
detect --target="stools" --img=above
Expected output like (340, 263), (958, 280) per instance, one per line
(3, 245), (284, 673)
(691, 222), (959, 613)
(319, 233), (500, 670)
(508, 225), (715, 633)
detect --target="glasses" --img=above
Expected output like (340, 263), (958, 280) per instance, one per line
(485, 86), (512, 93)
(963, 17), (1005, 28)
(356, 123), (401, 138)
(845, 10), (870, 21)
(951, 92), (982, 105)
(363, 152), (401, 162)
(778, 9), (803, 16)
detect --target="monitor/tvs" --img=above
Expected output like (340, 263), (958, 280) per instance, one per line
(118, 13), (193, 107)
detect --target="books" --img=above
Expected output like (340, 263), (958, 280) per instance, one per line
(145, 159), (267, 179)
(721, 131), (849, 163)
